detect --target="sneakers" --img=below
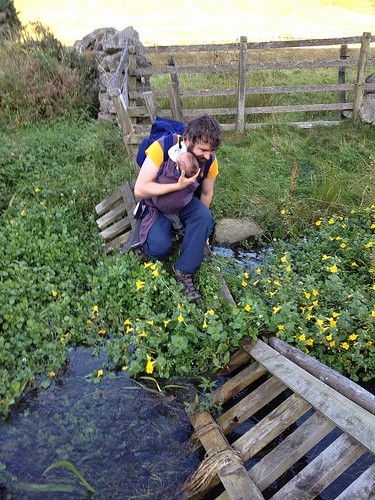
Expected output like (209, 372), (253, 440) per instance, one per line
(175, 268), (201, 300)
(135, 247), (148, 259)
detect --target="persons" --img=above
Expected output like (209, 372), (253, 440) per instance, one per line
(144, 151), (201, 215)
(134, 113), (223, 302)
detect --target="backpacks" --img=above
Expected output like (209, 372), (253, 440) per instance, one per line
(136, 117), (215, 184)
